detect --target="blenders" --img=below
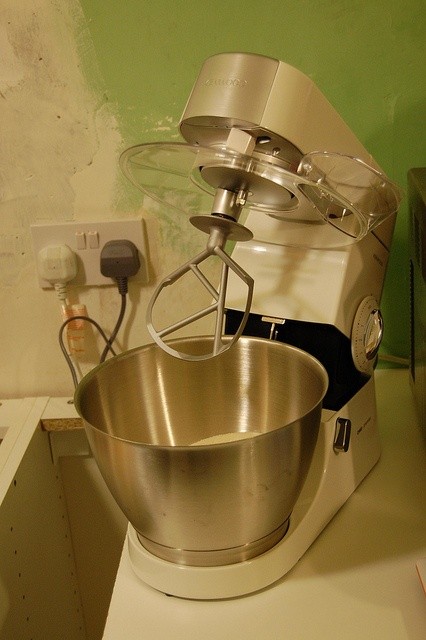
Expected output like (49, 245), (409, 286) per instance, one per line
(73, 50), (404, 600)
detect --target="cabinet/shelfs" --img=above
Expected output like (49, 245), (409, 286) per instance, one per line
(0, 396), (86, 640)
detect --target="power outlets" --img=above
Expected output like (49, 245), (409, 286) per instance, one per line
(28, 216), (151, 290)
